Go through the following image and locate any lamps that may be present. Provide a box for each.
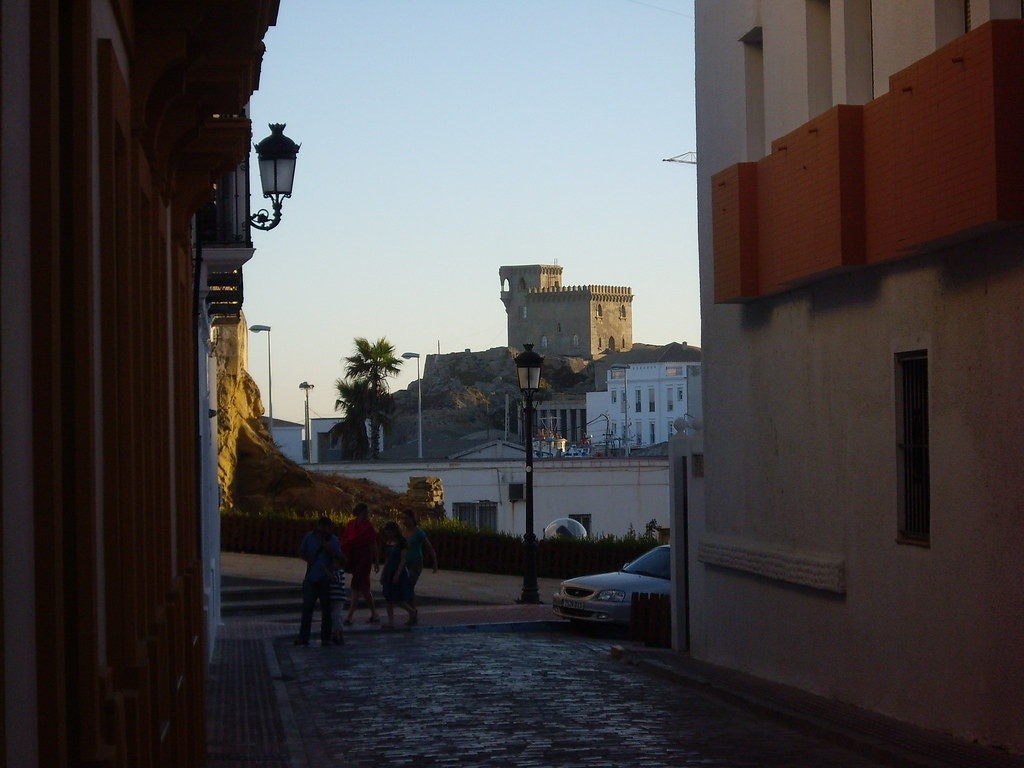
[250,122,300,231]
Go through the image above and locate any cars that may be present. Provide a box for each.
[552,543,670,639]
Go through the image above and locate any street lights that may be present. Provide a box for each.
[248,324,274,439]
[299,381,315,463]
[400,352,422,459]
[514,342,544,602]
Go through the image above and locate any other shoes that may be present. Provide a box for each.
[404,619,418,625]
[410,610,417,619]
[322,639,333,646]
[331,635,344,645]
[294,636,309,645]
[367,615,381,623]
[344,618,353,626]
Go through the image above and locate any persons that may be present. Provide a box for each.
[320,556,352,646]
[379,509,437,629]
[292,516,341,646]
[341,503,379,627]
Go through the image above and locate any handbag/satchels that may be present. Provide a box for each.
[423,551,432,569]
